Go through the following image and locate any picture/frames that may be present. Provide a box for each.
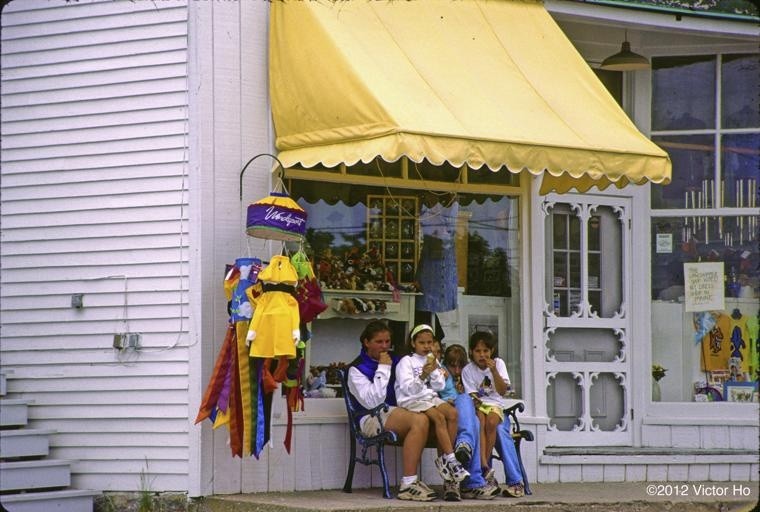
[723,382,759,403]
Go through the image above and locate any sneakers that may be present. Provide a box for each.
[396,481,438,502]
[461,485,501,500]
[483,467,498,487]
[434,440,473,481]
[443,482,462,501]
[502,482,526,498]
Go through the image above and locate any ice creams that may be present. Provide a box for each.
[427,353,435,363]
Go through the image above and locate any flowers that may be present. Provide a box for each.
[652,363,668,382]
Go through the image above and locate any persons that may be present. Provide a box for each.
[431,338,501,500]
[443,344,470,500]
[495,413,525,497]
[394,325,471,483]
[461,331,511,480]
[345,321,461,502]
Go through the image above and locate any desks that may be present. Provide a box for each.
[305,288,423,389]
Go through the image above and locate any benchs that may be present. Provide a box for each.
[337,368,534,499]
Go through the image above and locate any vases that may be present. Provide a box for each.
[652,383,660,401]
